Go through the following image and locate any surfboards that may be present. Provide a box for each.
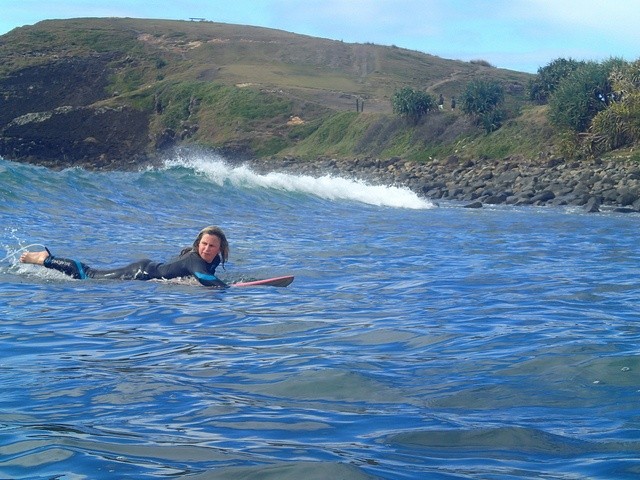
[229,276,295,287]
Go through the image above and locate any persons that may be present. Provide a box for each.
[20,225,233,290]
